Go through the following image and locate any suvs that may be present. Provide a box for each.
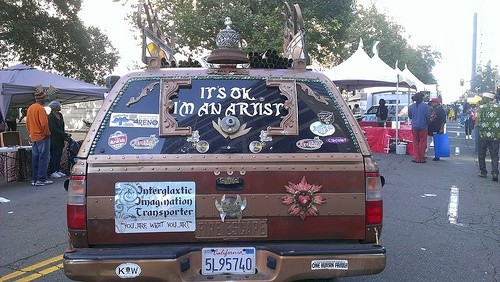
[61,68,387,282]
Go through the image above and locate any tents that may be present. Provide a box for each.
[0,63,116,184]
[319,37,439,99]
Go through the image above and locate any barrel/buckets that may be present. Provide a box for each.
[396,145,407,155]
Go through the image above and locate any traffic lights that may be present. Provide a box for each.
[460,78,465,86]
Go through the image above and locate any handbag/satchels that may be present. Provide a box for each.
[434,132,450,156]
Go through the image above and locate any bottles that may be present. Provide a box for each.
[383,121,386,128]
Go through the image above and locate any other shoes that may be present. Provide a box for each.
[433,157,439,161]
[466,135,468,139]
[468,135,472,139]
[478,170,487,178]
[32,181,44,185]
[492,174,498,181]
[411,159,426,163]
[36,180,53,184]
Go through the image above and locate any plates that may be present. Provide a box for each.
[310,121,336,138]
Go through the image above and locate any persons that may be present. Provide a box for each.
[444,102,463,121]
[427,97,447,136]
[48,101,71,179]
[474,91,491,154]
[25,85,54,186]
[464,101,473,140]
[377,99,388,125]
[408,91,430,164]
[476,89,500,181]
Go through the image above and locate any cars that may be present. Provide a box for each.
[362,104,410,124]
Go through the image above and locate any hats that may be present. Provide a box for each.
[35,89,46,101]
[430,98,439,102]
[49,101,60,109]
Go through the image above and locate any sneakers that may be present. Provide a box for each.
[56,171,66,176]
[50,172,61,177]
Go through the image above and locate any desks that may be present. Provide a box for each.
[358,121,414,155]
[0,145,33,183]
